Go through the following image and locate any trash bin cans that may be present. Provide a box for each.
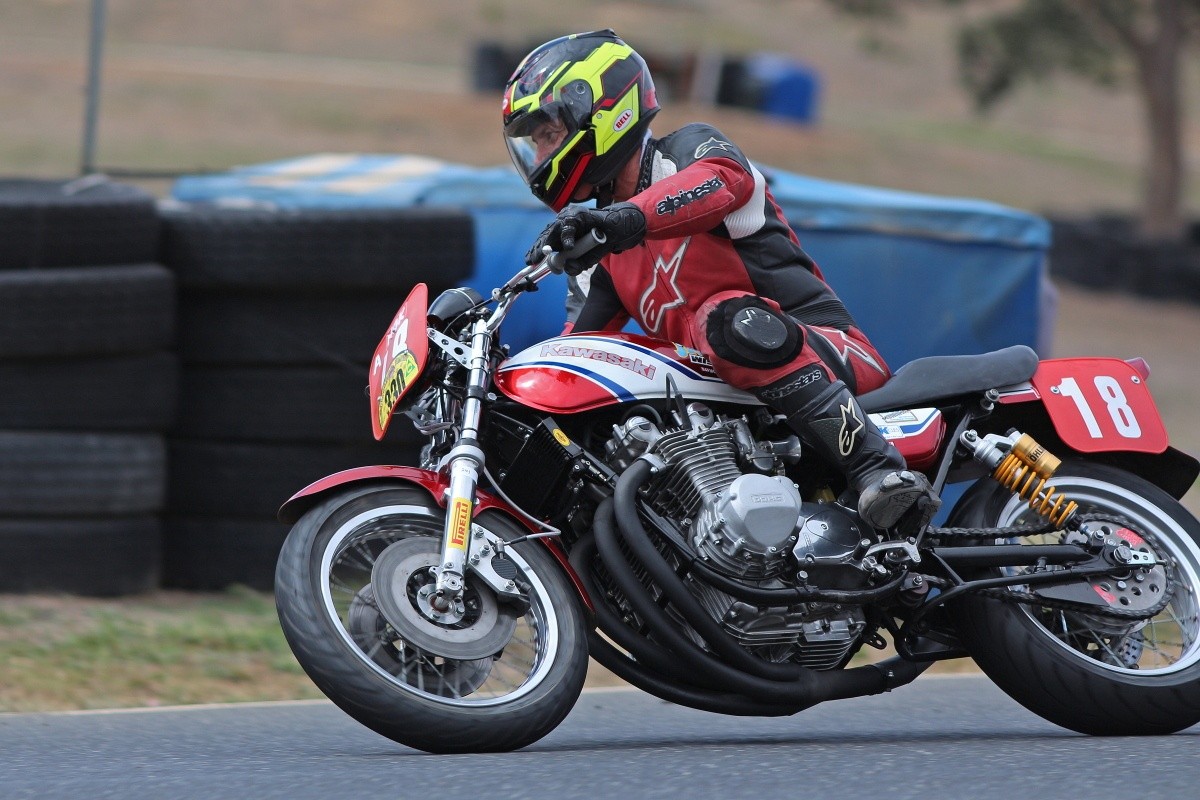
[720,53,815,118]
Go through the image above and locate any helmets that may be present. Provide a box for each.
[502,29,661,213]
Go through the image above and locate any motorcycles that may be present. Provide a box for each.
[274,226,1200,753]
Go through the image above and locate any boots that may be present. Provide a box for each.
[788,380,942,531]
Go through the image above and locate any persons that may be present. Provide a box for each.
[500,26,943,531]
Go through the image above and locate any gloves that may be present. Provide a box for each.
[525,201,645,276]
